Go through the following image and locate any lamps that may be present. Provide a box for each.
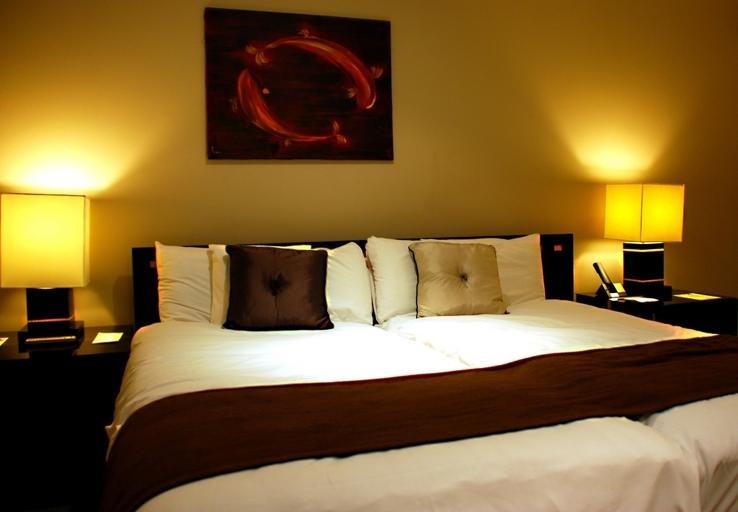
[603,183,685,301]
[1,193,90,343]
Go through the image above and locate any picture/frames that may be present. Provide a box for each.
[205,6,393,161]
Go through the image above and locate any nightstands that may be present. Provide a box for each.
[0,325,133,372]
[574,288,738,336]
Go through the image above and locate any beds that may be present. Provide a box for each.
[106,236,737,511]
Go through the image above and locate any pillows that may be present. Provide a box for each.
[208,242,373,327]
[407,241,510,318]
[365,233,546,324]
[155,239,312,323]
[221,245,335,330]
